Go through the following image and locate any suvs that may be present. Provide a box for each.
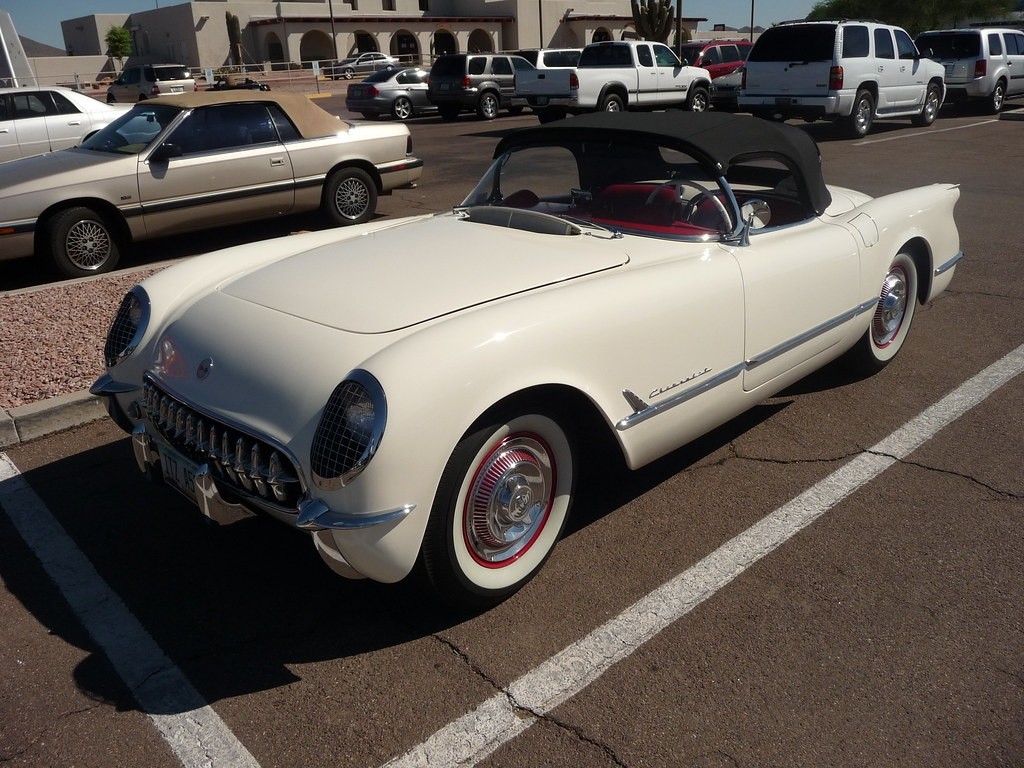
[668,38,755,106]
[735,16,948,138]
[105,62,199,103]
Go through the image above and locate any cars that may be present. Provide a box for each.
[204,77,272,92]
[0,86,164,164]
[344,65,440,120]
[323,52,400,81]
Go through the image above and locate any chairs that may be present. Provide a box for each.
[696,194,805,230]
[593,185,680,224]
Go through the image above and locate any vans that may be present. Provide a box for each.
[425,51,536,120]
[914,27,1024,116]
[513,45,584,69]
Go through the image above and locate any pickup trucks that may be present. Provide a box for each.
[512,40,714,125]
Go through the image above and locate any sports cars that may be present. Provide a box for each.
[1,81,427,279]
[87,108,966,613]
